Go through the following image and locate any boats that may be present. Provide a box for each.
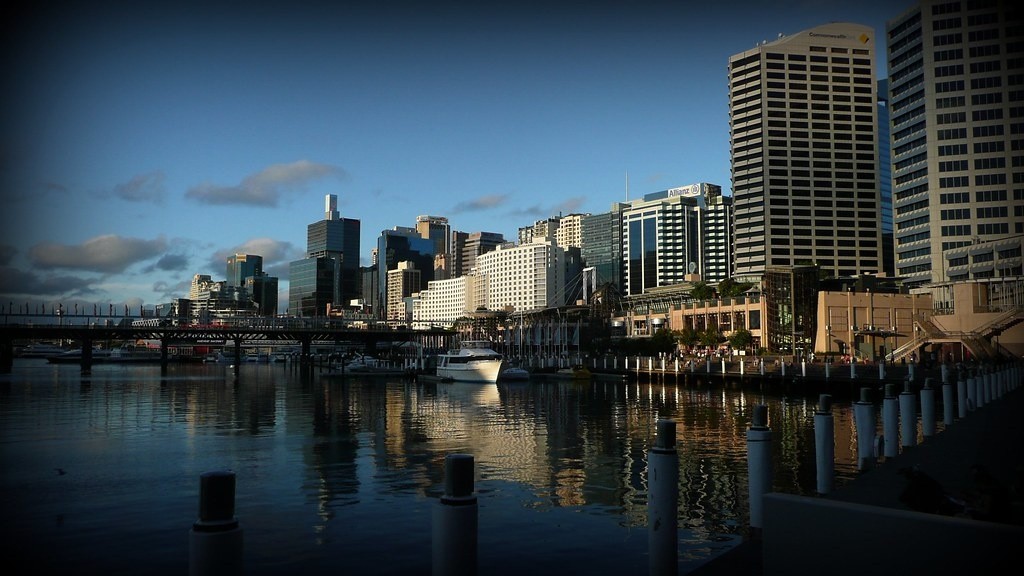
[247,352,289,362]
[47,348,119,364]
[502,367,530,379]
[436,341,502,383]
[557,364,591,378]
